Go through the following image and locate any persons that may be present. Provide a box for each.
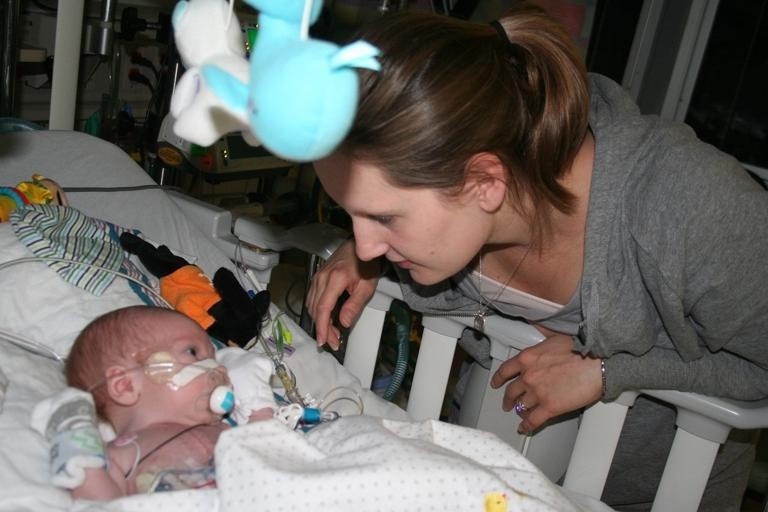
[37,304,281,503]
[299,1,765,510]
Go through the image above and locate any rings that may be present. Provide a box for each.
[512,398,529,418]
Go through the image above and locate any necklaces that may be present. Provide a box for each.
[471,241,533,341]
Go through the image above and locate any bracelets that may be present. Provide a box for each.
[599,354,607,398]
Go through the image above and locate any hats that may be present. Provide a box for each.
[10,202,125,298]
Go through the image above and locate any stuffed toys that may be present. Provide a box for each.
[167,0,262,151]
[201,0,386,165]
[0,173,68,226]
[120,231,273,350]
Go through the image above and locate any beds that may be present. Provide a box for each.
[0,121,768,511]
